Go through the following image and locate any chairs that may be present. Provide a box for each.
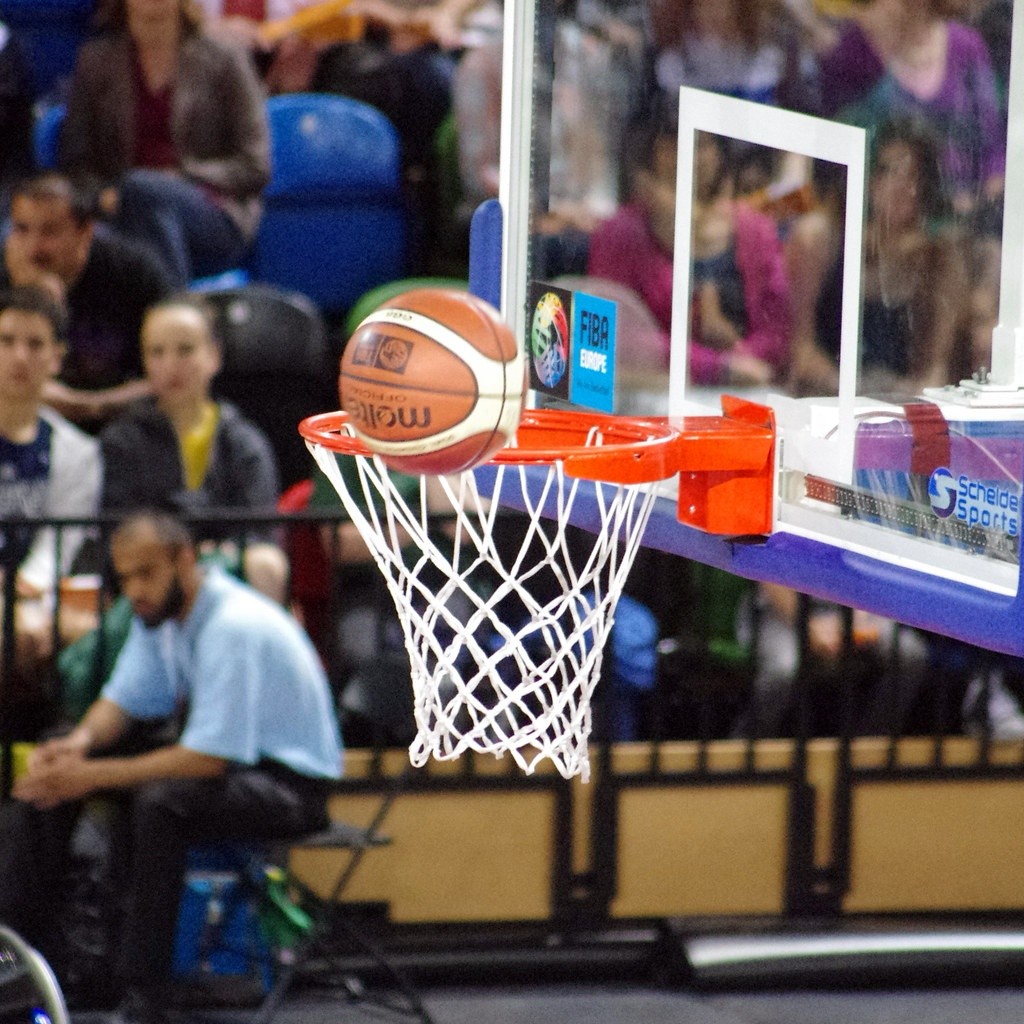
[206,289,324,488]
[175,652,463,1024]
[251,94,405,323]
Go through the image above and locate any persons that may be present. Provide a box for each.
[0,0,1006,1023]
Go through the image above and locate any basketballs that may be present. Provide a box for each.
[340,286,523,471]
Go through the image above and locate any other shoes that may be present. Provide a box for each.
[170,975,263,1009]
[68,1010,126,1024]
